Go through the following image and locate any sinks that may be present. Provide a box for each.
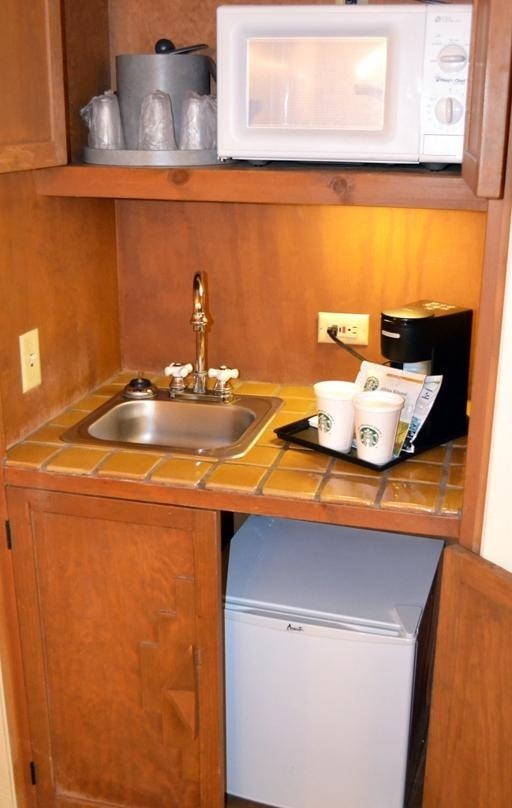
[61,387,283,460]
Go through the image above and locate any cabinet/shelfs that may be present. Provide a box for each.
[0,1,512,808]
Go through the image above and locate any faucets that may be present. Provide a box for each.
[190,270,209,397]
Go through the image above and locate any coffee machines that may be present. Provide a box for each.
[381,299,473,459]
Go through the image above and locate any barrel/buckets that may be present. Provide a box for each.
[115,43,208,152]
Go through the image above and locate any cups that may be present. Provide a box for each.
[88,96,124,149]
[313,380,360,454]
[352,389,405,465]
[137,96,176,150]
[180,98,212,150]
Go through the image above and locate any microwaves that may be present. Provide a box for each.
[215,4,473,165]
